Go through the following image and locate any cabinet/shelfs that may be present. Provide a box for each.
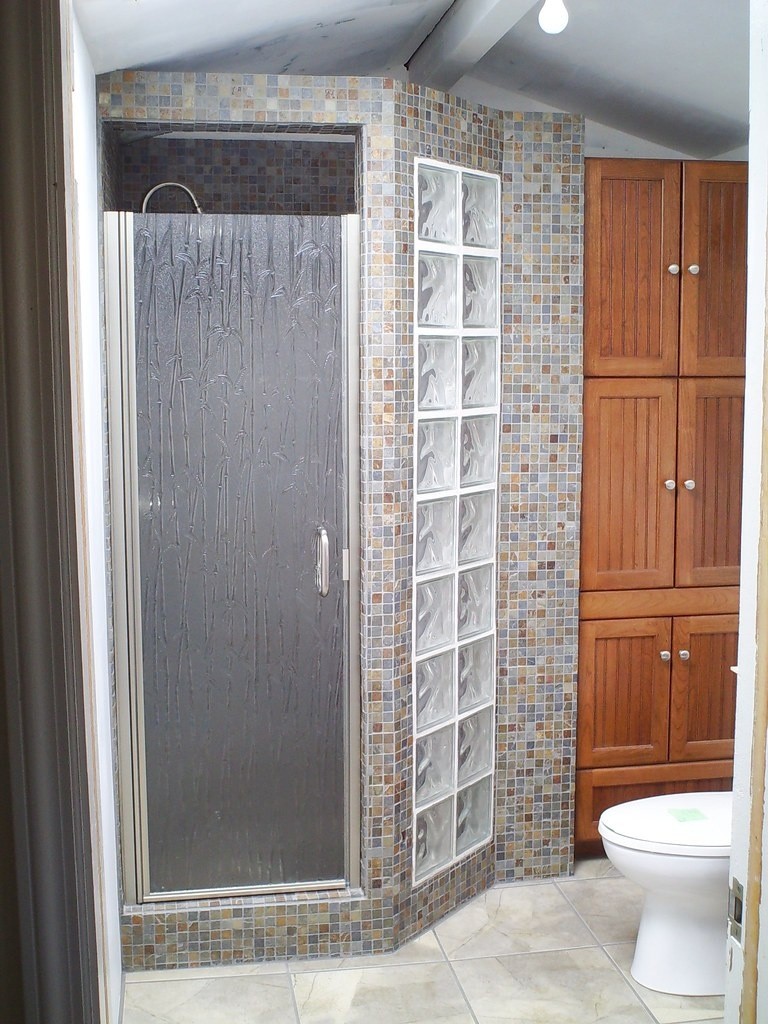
[573,158,749,862]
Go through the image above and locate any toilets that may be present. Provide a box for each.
[599,790,730,997]
